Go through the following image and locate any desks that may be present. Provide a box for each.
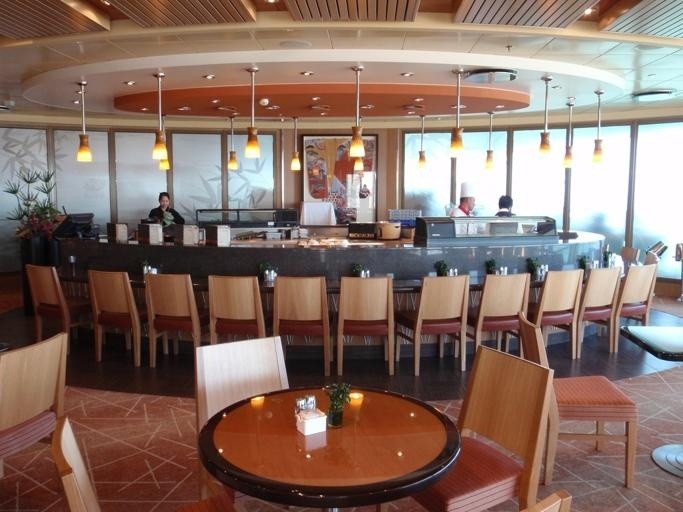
[197,385,462,512]
[620,325,683,478]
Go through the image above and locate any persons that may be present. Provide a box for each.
[450,192,477,234]
[149,192,185,225]
[489,195,518,234]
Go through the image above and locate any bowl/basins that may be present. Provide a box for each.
[521,224,536,233]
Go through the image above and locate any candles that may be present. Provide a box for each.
[251,396,265,405]
[347,392,364,406]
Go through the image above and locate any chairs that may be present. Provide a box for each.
[376,345,555,512]
[194,335,289,504]
[514,491,573,512]
[0,332,68,479]
[51,415,238,512]
[518,311,640,490]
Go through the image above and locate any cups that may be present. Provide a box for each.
[295,393,322,419]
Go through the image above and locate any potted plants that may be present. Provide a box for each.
[321,382,353,428]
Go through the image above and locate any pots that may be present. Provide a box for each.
[376,220,403,239]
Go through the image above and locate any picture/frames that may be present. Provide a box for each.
[300,133,378,227]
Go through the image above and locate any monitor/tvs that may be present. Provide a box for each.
[54,213,94,238]
[279,210,297,223]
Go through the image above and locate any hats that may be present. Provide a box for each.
[460,180,477,199]
